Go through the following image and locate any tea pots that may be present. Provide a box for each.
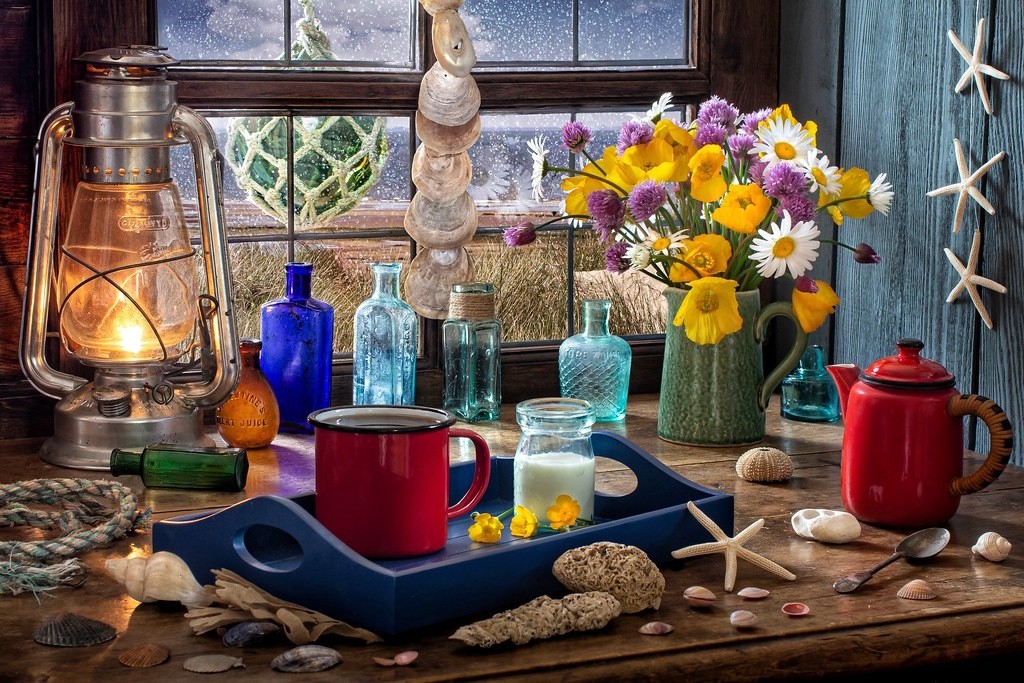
[826,339,1013,526]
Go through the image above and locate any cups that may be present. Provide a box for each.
[307,405,489,560]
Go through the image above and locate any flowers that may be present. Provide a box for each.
[510,506,554,539]
[468,509,514,543]
[545,494,593,533]
[502,91,895,346]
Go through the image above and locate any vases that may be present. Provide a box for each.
[438,282,502,421]
[557,300,634,422]
[656,287,809,448]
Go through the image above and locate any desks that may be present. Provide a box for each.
[0,395,1024,683]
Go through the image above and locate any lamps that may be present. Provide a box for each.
[17,44,243,472]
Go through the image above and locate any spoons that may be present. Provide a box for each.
[833,528,950,592]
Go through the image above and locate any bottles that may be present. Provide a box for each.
[261,262,334,435]
[514,398,595,532]
[558,300,631,420]
[110,443,248,490]
[780,345,841,421]
[443,283,502,422]
[352,264,417,404]
[215,339,280,449]
